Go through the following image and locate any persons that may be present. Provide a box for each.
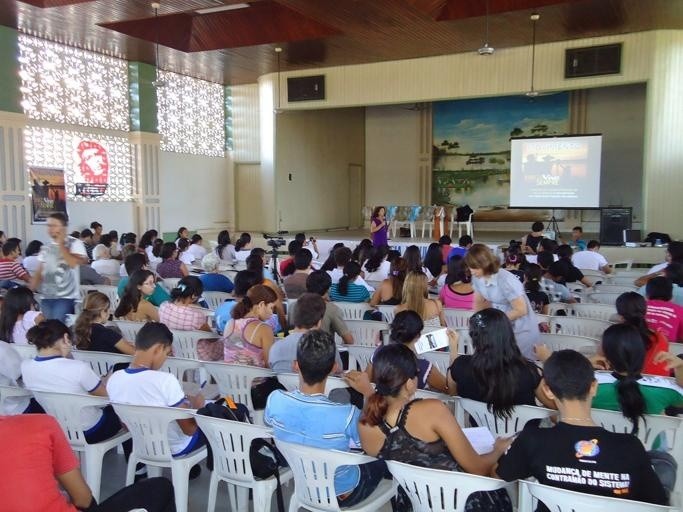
[77,141,109,193]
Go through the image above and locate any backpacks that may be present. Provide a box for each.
[363,310,389,340]
[196,397,280,480]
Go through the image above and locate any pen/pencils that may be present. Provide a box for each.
[508,431,521,439]
[198,380,207,393]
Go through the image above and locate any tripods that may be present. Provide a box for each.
[269,254,284,283]
[544,215,562,238]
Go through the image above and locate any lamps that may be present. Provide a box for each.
[526,15,541,98]
[477,1,495,57]
[273,47,285,115]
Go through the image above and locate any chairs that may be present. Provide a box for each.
[370,203,474,240]
[0,239,683,512]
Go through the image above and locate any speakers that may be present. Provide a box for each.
[599,206,632,246]
[287,75,325,102]
[564,42,622,78]
